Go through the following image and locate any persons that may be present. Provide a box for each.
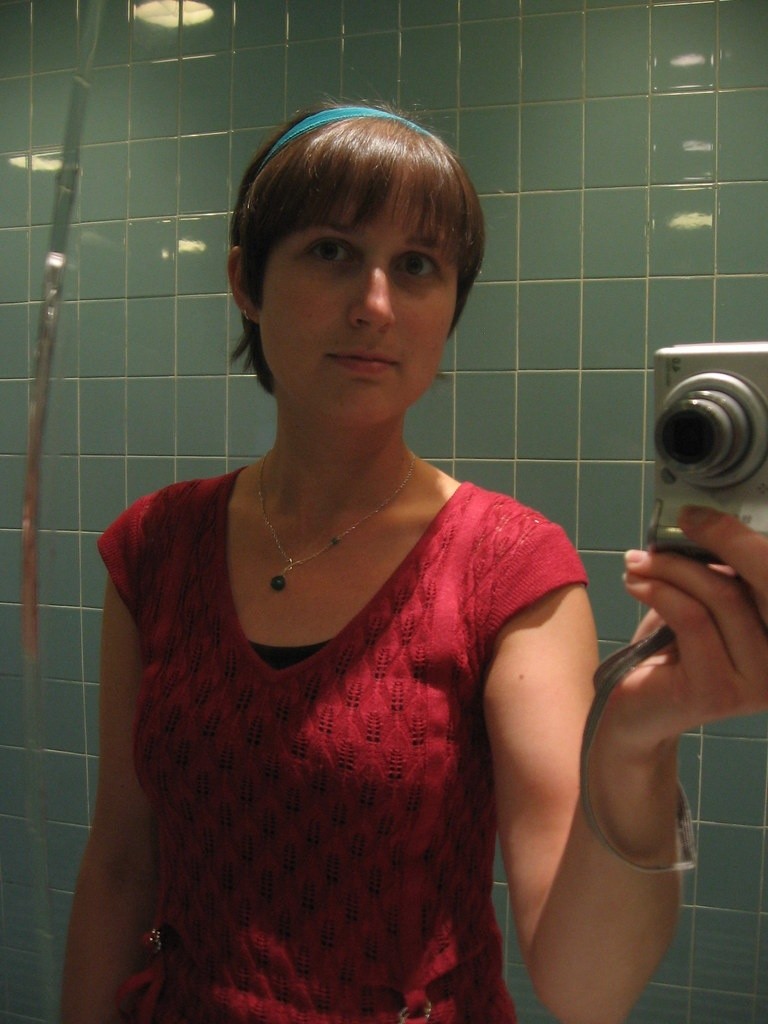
[62,96,766,1021]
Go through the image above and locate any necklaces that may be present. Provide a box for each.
[253,444,417,591]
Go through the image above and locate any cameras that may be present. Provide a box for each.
[645,340,768,552]
